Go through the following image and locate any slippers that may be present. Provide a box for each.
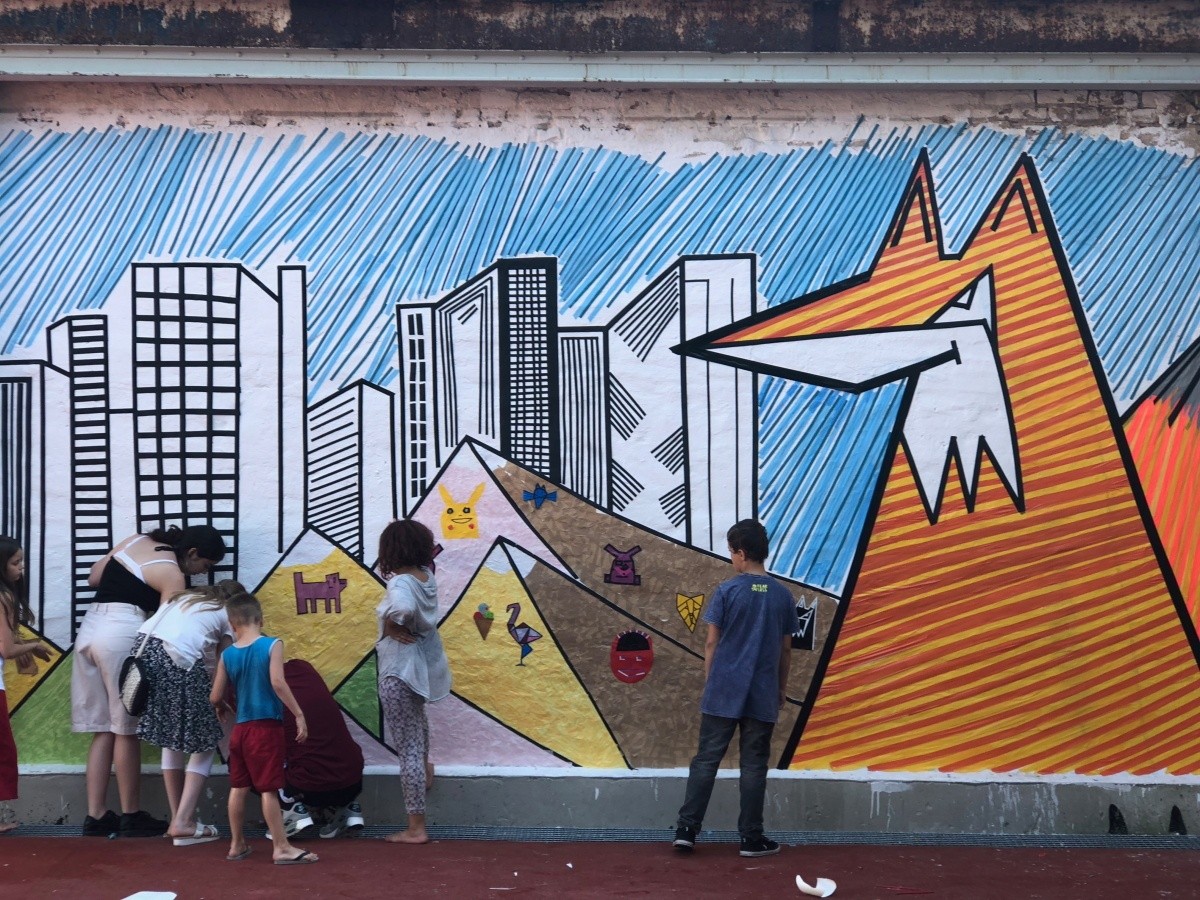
[227,845,252,860]
[173,822,220,846]
[275,850,317,864]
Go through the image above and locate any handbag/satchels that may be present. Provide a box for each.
[118,656,151,717]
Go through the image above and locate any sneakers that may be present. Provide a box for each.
[319,801,364,839]
[739,834,780,856]
[82,809,120,836]
[672,826,695,847]
[266,802,314,840]
[120,810,170,837]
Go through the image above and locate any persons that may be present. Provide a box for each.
[672,519,800,857]
[132,579,246,846]
[209,594,319,865]
[70,525,227,839]
[0,535,54,831]
[374,520,451,844]
[261,659,365,841]
